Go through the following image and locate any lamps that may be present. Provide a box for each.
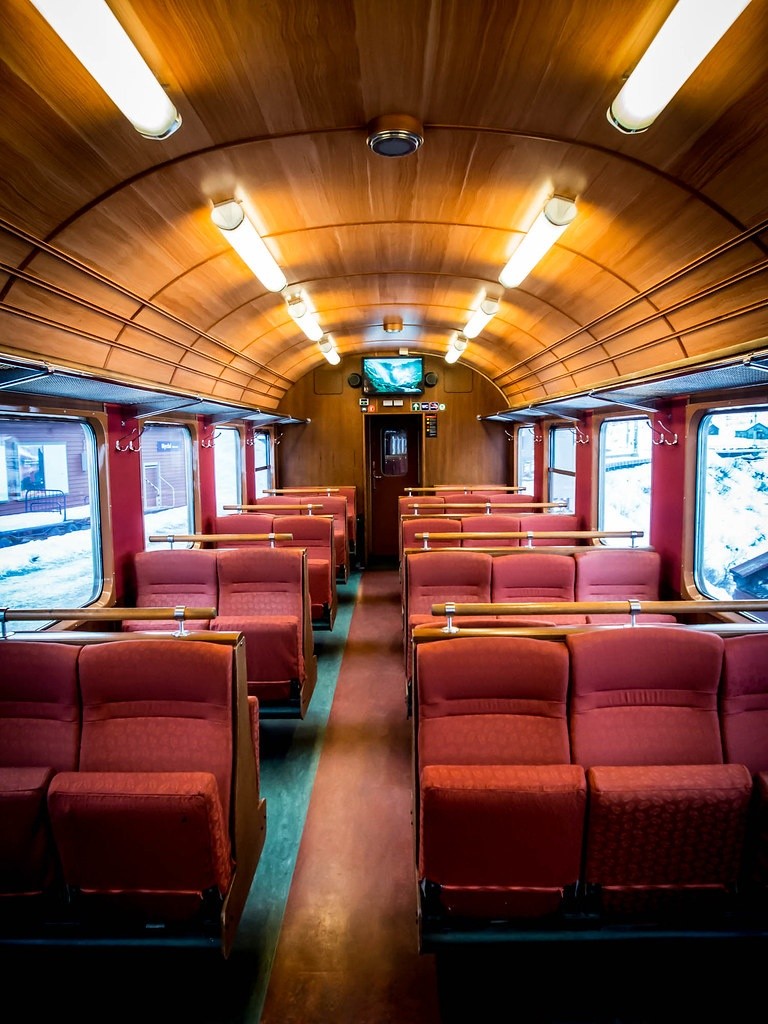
[29,0,183,141]
[288,298,323,341]
[498,194,577,289]
[606,0,752,135]
[319,339,341,366]
[445,336,467,364]
[211,202,288,293]
[463,296,499,339]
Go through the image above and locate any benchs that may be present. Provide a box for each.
[0,484,357,959]
[397,485,768,955]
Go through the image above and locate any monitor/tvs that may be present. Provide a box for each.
[361,356,424,395]
